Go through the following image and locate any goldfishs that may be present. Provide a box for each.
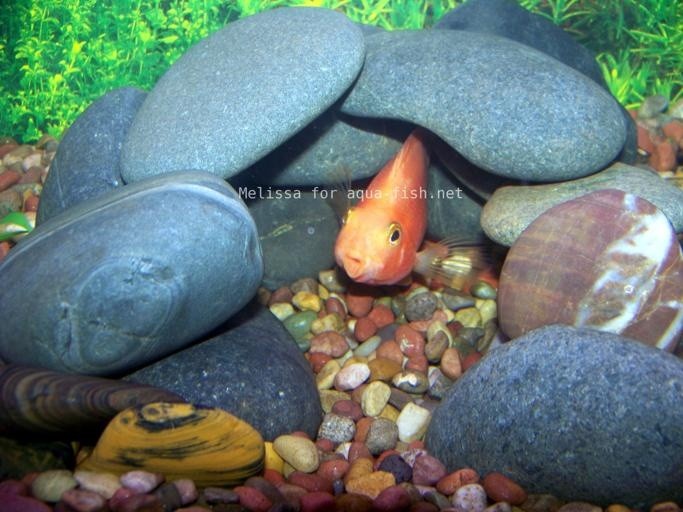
[317,126,508,292]
